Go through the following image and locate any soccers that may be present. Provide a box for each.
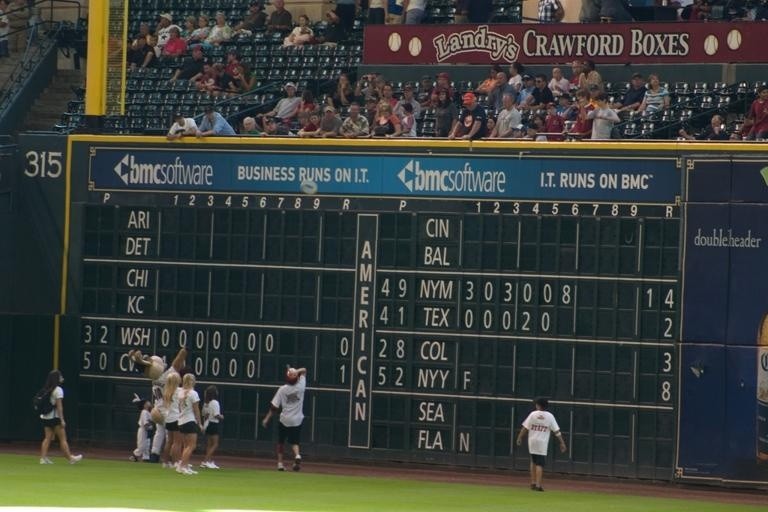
[727,30,743,50]
[704,35,719,55]
[408,38,421,56]
[388,33,402,51]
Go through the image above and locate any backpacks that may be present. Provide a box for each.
[33,386,54,415]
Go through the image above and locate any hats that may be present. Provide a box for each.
[160,10,309,88]
[48,370,64,384]
[280,69,584,119]
[287,368,297,379]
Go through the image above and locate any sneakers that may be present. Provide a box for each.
[151,455,160,463]
[142,459,150,462]
[69,453,83,465]
[39,458,54,465]
[278,462,288,472]
[162,460,198,476]
[293,455,302,472]
[128,455,139,462]
[200,461,219,469]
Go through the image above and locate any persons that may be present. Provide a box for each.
[129,11,232,72]
[171,45,258,102]
[261,366,307,471]
[37,369,83,465]
[167,107,235,140]
[234,0,356,53]
[579,0,767,23]
[244,73,435,137]
[609,73,670,135]
[515,396,567,491]
[678,87,767,141]
[368,0,494,24]
[0,0,31,65]
[538,0,564,24]
[122,346,227,474]
[430,60,619,142]
